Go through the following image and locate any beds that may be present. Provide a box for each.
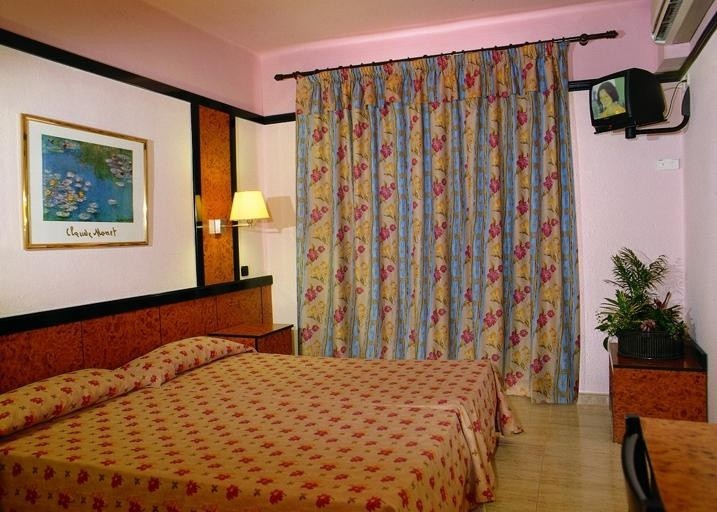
[0,335,520,511]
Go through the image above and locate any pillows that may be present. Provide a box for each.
[114,335,254,388]
[0,369,137,436]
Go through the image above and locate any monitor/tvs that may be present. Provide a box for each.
[590,68,665,126]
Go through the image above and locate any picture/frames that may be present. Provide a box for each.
[20,113,150,249]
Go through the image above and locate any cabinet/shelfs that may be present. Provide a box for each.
[607,342,708,445]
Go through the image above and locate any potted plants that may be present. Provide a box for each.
[594,245,688,360]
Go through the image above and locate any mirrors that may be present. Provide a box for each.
[191,102,241,286]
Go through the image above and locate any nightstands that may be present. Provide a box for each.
[210,323,294,354]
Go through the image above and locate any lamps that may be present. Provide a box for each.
[215,190,272,236]
[195,196,216,234]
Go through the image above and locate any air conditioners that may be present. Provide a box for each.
[650,0,715,44]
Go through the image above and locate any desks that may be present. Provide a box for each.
[641,416,717,512]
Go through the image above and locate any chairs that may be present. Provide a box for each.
[621,416,658,512]
